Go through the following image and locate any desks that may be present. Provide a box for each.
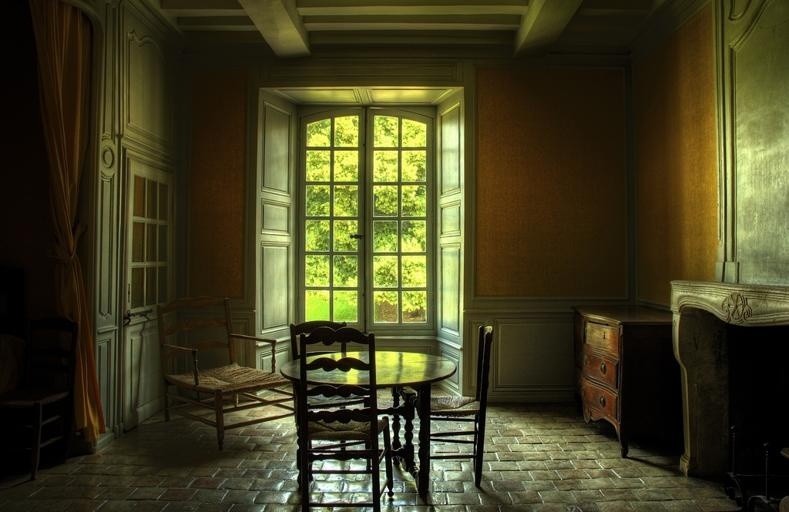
[280,350,457,496]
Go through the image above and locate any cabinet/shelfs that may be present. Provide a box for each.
[567,304,684,459]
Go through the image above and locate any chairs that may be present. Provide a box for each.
[156,295,297,450]
[289,320,495,512]
[1,310,77,480]
